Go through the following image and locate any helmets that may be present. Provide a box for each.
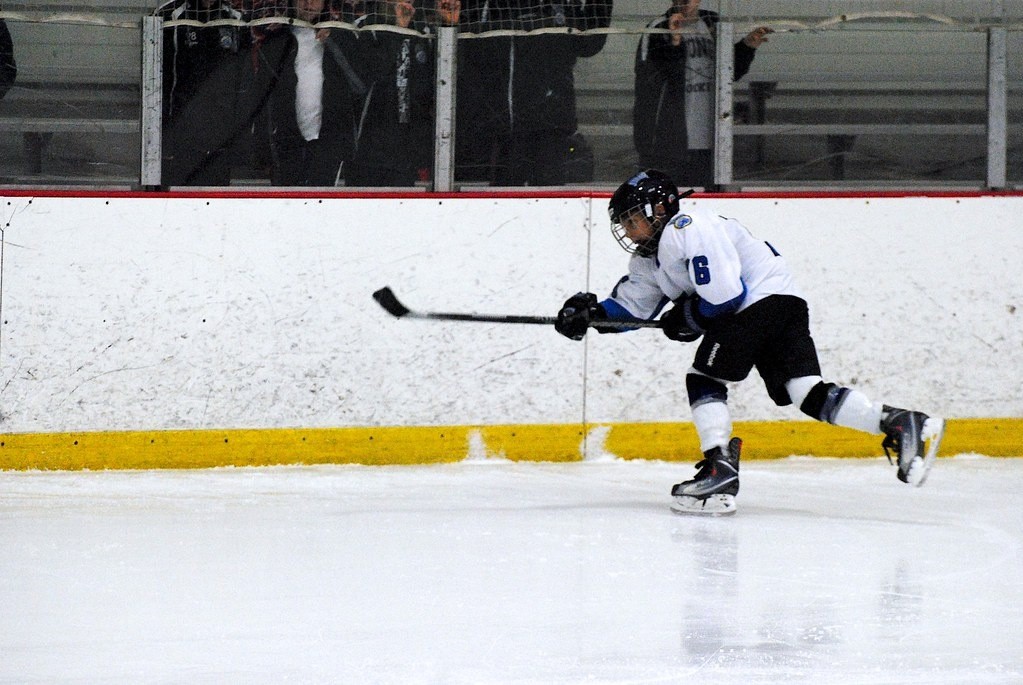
[609,169,680,259]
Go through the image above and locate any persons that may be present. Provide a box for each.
[633,1,773,185]
[146,0,614,188]
[553,167,945,518]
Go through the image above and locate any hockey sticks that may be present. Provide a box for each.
[371,285,663,329]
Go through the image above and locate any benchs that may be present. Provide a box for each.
[0,0,1023,137]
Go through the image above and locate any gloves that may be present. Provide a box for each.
[661,292,706,342]
[556,293,597,341]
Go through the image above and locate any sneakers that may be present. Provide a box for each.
[880,404,946,487]
[671,438,743,516]
